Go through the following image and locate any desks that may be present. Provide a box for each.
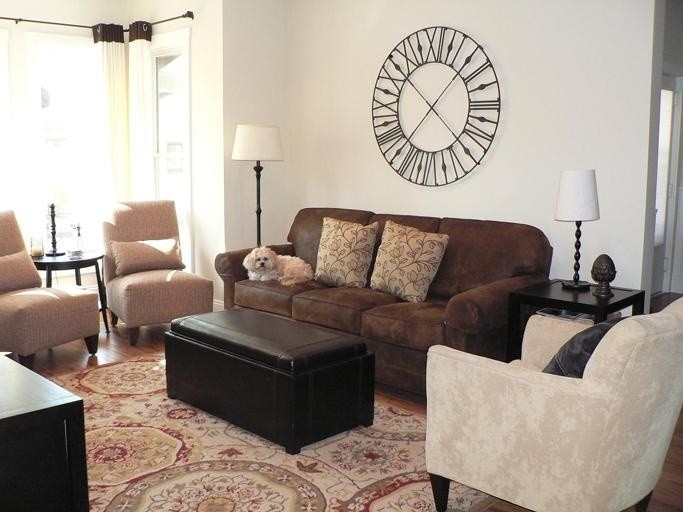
[505,274,645,365]
[162,307,374,457]
[32,248,112,335]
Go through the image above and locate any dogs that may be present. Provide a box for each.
[242,245,315,286]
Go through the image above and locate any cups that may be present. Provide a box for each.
[31,235,44,257]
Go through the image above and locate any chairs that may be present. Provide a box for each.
[421,292,682,510]
[0,202,99,367]
[95,196,215,350]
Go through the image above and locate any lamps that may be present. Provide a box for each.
[555,164,602,291]
[229,121,284,247]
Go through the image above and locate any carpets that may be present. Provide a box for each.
[40,344,511,511]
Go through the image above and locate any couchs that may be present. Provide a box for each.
[215,202,551,405]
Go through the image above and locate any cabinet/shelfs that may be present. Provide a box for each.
[0,350,93,512]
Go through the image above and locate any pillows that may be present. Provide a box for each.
[370,216,450,302]
[0,247,42,293]
[543,316,627,379]
[109,237,185,274]
[312,216,381,289]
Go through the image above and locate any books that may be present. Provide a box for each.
[536,307,583,322]
